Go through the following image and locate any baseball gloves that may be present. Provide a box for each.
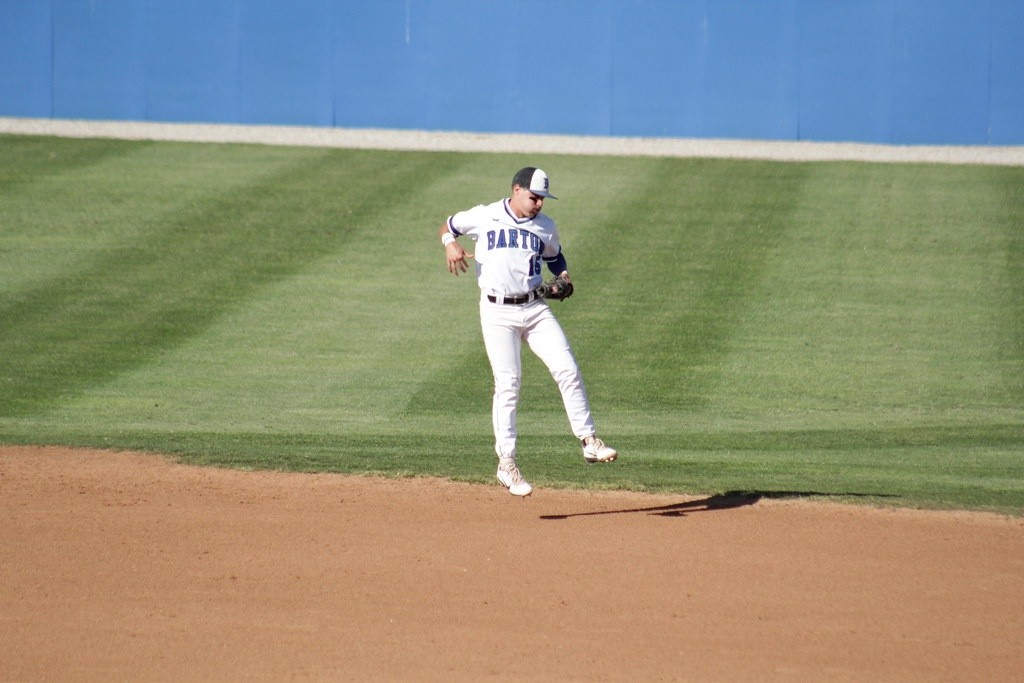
[538,277,574,302]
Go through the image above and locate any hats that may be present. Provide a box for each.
[512,167,560,201]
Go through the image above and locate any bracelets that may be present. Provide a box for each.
[441,232,455,246]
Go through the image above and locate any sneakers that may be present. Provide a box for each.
[496,463,533,498]
[582,438,618,464]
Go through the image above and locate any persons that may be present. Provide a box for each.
[439,166,618,498]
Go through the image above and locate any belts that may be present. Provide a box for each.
[488,291,539,304]
